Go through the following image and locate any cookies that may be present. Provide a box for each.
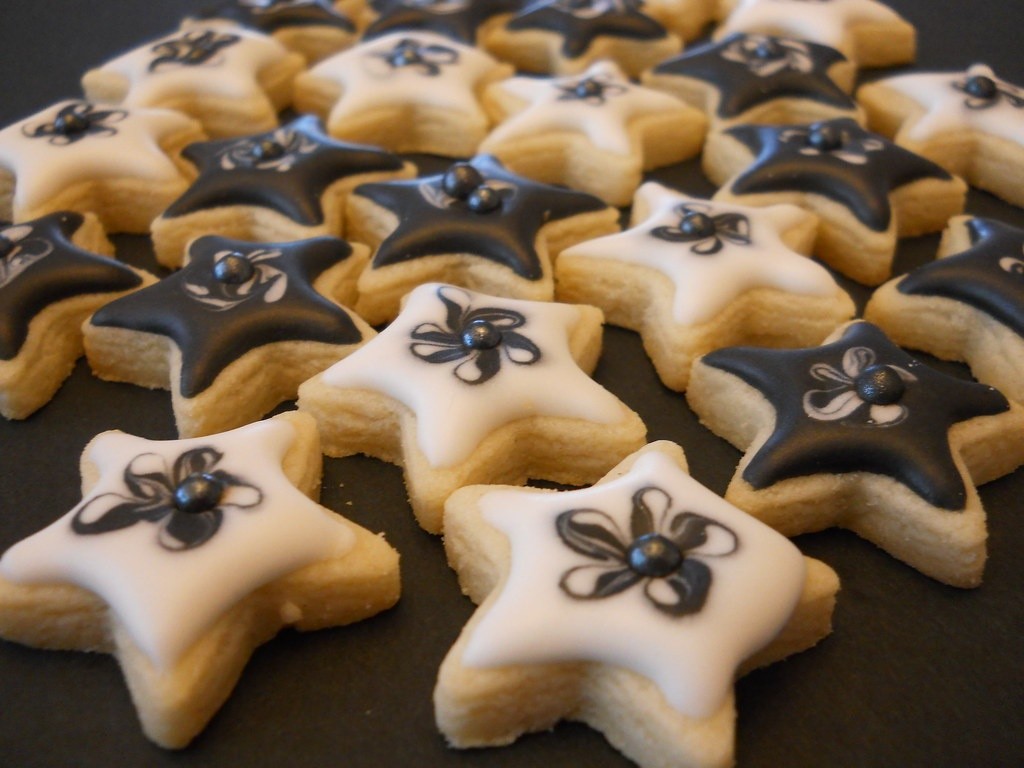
[0,0,1024,768]
